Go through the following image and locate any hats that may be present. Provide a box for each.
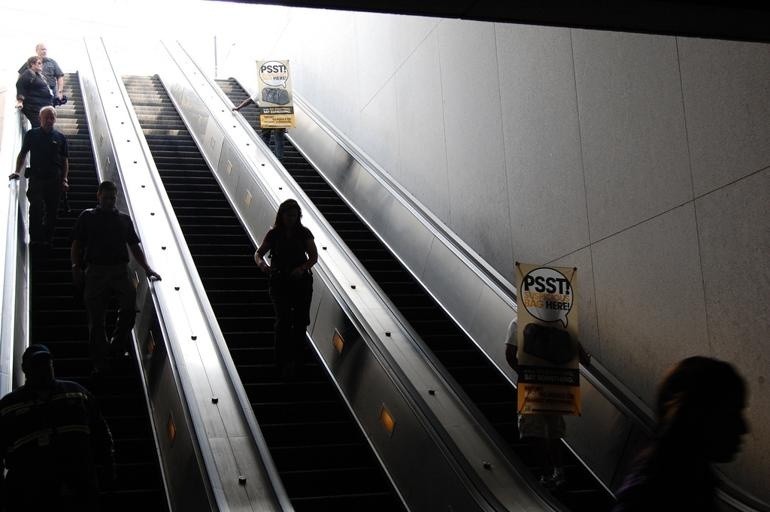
[21,340,56,366]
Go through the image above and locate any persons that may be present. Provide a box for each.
[9,106,70,244]
[254,199,317,366]
[0,344,113,512]
[616,356,749,512]
[232,97,284,161]
[15,43,64,128]
[71,181,162,357]
[505,316,591,486]
